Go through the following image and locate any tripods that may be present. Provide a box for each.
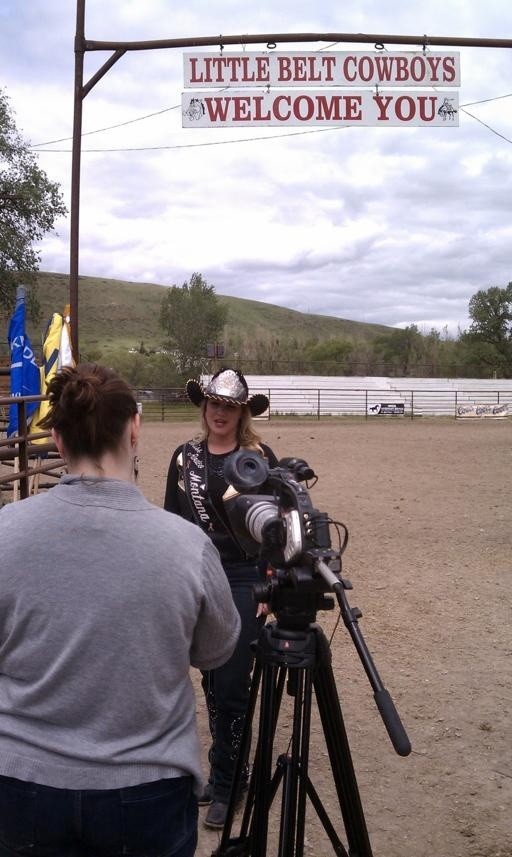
[212,548,412,857]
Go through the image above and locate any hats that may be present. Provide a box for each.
[186,367,269,417]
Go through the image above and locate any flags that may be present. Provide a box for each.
[27,310,63,446]
[6,284,42,452]
[56,303,76,375]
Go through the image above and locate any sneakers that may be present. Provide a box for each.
[196,783,243,828]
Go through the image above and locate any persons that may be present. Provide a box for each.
[0,363,243,855]
[161,362,281,835]
